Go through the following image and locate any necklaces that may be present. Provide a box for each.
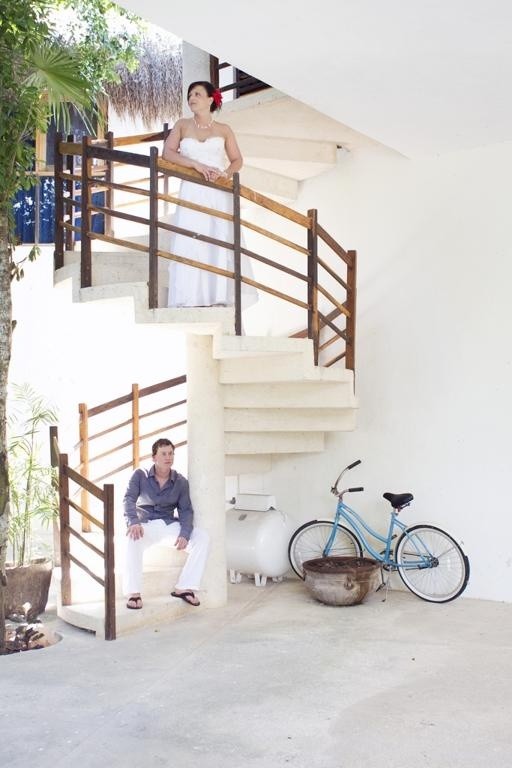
[192,117,213,131]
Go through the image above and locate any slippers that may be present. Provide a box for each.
[127,596,143,609]
[171,591,201,606]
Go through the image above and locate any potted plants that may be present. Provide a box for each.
[4,379,62,620]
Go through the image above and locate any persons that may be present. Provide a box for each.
[161,81,245,307]
[124,439,201,609]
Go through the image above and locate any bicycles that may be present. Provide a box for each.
[287,458,470,602]
